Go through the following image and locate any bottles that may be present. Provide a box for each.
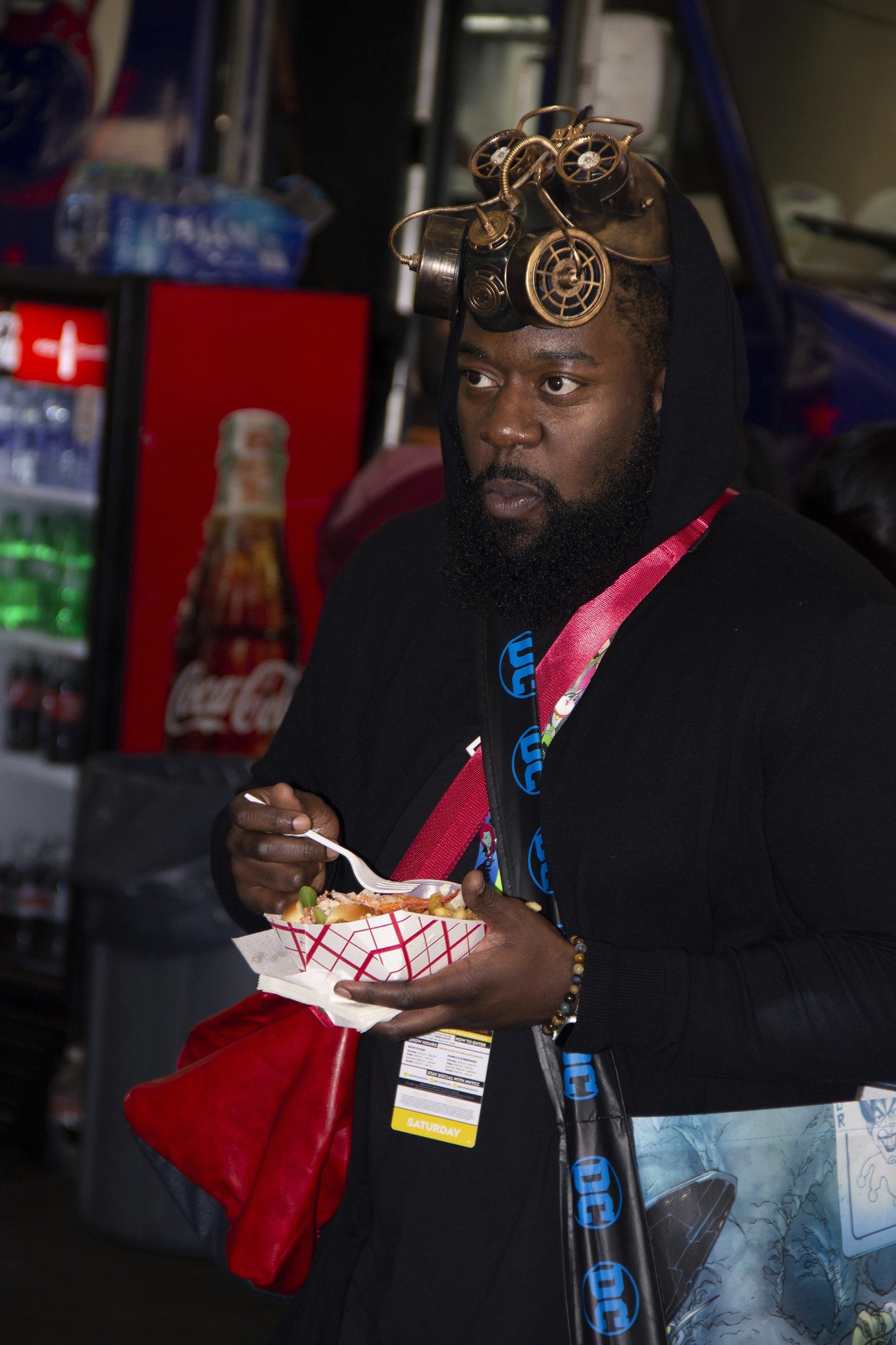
[164,408,304,757]
[1,383,104,1199]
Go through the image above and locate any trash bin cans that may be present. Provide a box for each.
[72,753,259,1254]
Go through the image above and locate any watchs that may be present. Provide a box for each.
[552,1000,580,1049]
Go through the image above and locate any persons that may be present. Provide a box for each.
[210,151,896,1345]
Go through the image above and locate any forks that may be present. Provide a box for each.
[244,793,420,893]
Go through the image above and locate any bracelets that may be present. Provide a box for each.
[541,933,587,1034]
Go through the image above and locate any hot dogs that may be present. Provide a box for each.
[279,886,428,924]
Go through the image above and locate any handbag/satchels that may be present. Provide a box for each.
[124,989,360,1296]
